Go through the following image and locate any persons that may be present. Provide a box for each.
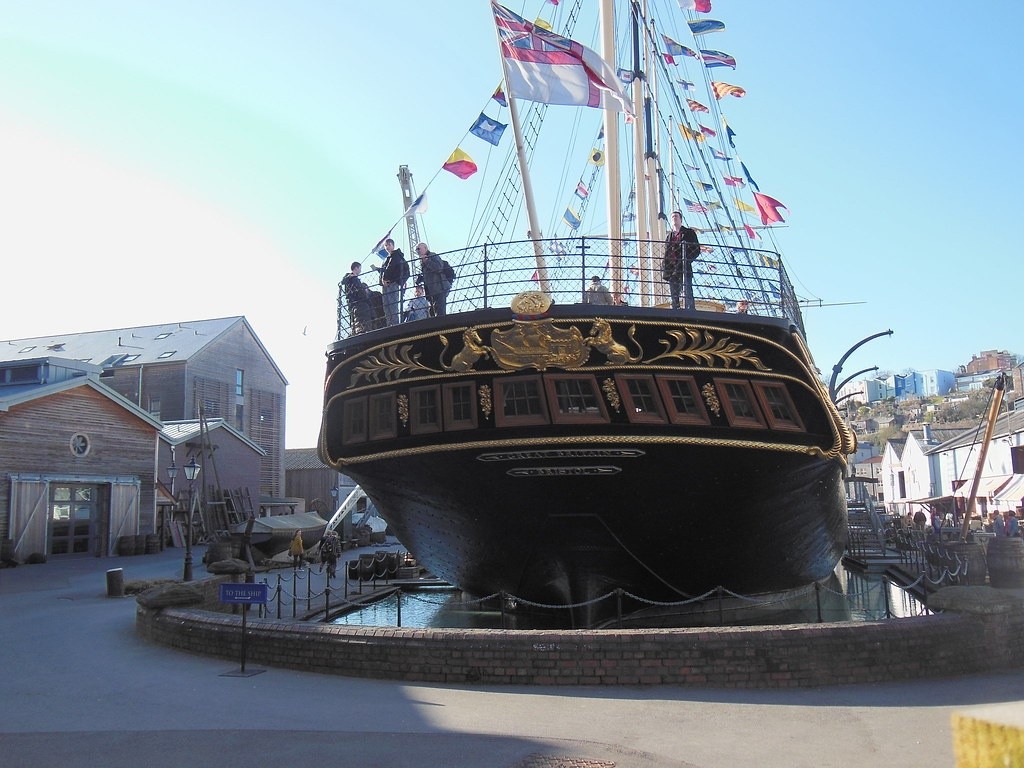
[292,528,304,571]
[376,239,404,326]
[406,286,429,321]
[586,276,613,305]
[902,508,940,542]
[994,510,1018,537]
[318,531,341,578]
[341,262,386,331]
[661,211,700,309]
[415,242,452,316]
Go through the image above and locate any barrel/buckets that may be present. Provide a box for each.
[120,533,161,557]
[208,542,232,567]
[946,544,986,585]
[985,537,1024,588]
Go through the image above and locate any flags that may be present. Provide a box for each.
[491,2,633,125]
[661,0,787,298]
[546,0,559,6]
[469,112,509,147]
[535,17,551,31]
[617,68,632,83]
[443,148,477,180]
[622,192,639,294]
[405,194,428,216]
[373,234,391,260]
[492,86,508,107]
[531,128,604,284]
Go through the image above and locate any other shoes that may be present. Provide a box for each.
[330,572,336,579]
[319,566,323,573]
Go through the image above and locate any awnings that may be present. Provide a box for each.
[889,474,1024,512]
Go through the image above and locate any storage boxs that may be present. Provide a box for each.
[398,566,419,579]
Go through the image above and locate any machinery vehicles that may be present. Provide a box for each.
[396,165,429,296]
[937,371,1013,544]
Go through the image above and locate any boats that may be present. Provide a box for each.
[228,511,329,555]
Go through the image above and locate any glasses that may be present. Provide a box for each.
[416,248,421,251]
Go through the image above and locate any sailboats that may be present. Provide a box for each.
[315,0,858,620]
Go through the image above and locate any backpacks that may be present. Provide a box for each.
[322,536,335,557]
[396,257,411,284]
[432,255,457,285]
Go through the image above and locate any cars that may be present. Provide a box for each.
[846,507,871,532]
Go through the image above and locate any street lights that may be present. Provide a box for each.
[182,454,202,581]
[330,486,338,514]
[166,460,182,522]
